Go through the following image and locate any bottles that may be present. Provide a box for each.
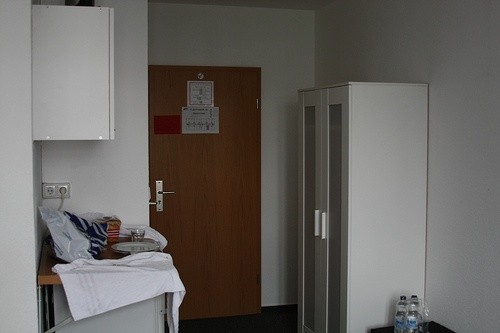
[394,296,408,333]
[406,296,420,333]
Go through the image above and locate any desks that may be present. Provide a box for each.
[39,233,183,333]
[371,321,457,333]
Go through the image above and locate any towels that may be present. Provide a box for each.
[51,251,187,333]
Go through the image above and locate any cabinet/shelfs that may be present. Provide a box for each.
[31,5,116,142]
[295,80,430,333]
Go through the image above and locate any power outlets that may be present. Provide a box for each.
[42,183,69,198]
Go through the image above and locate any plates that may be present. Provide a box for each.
[111,242,157,252]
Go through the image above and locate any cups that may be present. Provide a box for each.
[131,230,144,242]
